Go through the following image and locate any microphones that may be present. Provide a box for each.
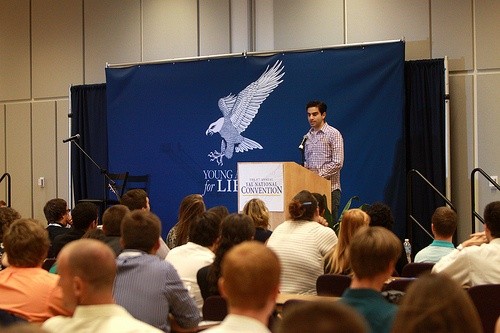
[299,135,308,150]
[63,133,81,143]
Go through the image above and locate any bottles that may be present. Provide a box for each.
[403,239,411,263]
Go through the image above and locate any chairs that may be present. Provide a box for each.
[0,247,499,333]
[77,172,149,224]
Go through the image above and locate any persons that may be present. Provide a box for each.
[431,201,500,289]
[324,203,395,277]
[112,210,201,333]
[164,194,273,320]
[41,238,165,333]
[0,206,74,325]
[196,240,281,333]
[413,206,461,264]
[241,190,338,296]
[273,226,403,333]
[392,271,481,333]
[42,189,171,274]
[302,100,344,226]
